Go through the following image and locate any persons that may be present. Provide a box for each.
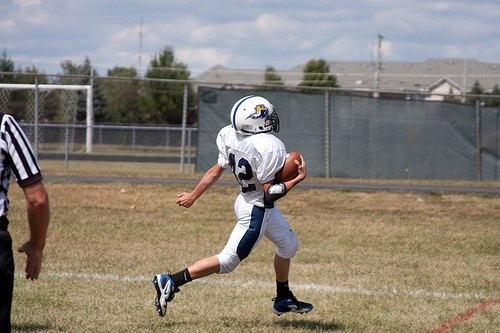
[0,113,50,333]
[153,95,314,317]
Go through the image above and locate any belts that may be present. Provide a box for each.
[0,217,8,231]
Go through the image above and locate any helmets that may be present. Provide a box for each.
[230,95,274,135]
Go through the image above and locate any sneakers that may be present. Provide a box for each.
[151,270,180,317]
[272,291,313,316]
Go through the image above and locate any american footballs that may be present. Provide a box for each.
[273,151,302,182]
